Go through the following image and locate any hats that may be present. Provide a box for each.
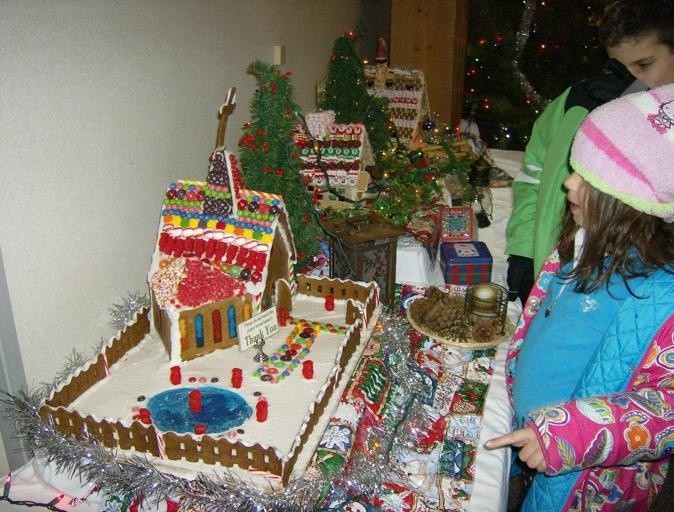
[569,83,674,223]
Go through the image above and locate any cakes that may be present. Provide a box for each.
[35,67,449,491]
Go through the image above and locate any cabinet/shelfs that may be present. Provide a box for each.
[389,2,468,133]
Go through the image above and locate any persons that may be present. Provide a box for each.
[501,57,655,300]
[481,76,674,512]
[598,0,674,92]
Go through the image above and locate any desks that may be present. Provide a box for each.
[2,140,525,512]
